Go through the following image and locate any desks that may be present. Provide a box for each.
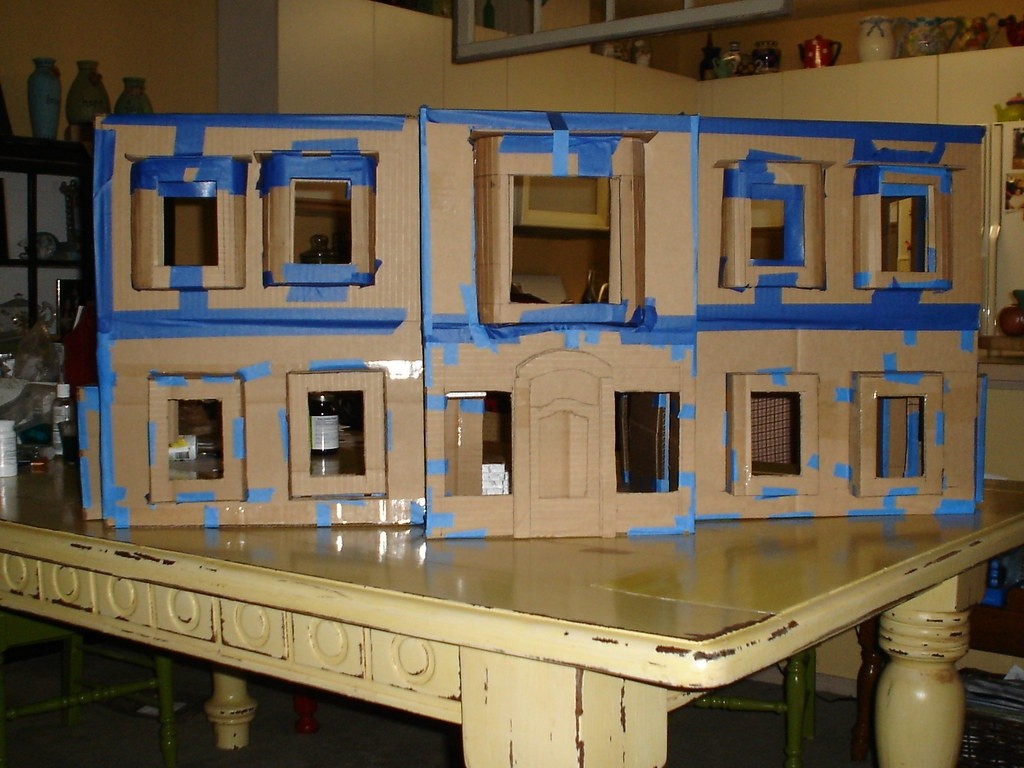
[0,451,1024,768]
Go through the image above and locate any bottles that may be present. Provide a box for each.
[0,420,17,477]
[52,384,76,456]
[66,59,107,123]
[700,47,721,80]
[309,234,331,264]
[114,76,153,114]
[310,396,340,453]
[28,57,61,139]
[722,41,743,77]
[752,40,780,74]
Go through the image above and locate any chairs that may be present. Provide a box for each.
[850,543,1024,763]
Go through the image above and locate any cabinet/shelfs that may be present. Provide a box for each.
[216,0,1024,125]
[979,363,1022,483]
[0,133,93,344]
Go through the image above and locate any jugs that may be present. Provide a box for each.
[902,13,1024,56]
[857,15,909,60]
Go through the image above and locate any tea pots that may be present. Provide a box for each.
[994,93,1024,122]
[798,35,841,68]
